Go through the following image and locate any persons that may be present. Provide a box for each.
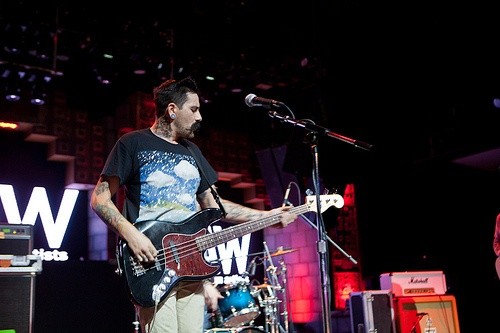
[202,223,263,329]
[90,77,297,333]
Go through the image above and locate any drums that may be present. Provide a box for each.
[203,328,236,333]
[237,327,268,333]
[213,284,261,328]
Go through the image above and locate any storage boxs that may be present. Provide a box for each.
[0,272,36,333]
[348,289,395,333]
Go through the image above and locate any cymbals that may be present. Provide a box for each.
[254,283,280,290]
[262,247,300,259]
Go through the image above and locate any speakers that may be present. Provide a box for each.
[391,295,461,333]
[0,272,33,333]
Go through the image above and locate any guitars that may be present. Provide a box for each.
[116,188,344,308]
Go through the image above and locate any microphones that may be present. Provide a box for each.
[242,256,256,279]
[244,93,283,108]
[282,186,291,207]
[416,313,428,316]
